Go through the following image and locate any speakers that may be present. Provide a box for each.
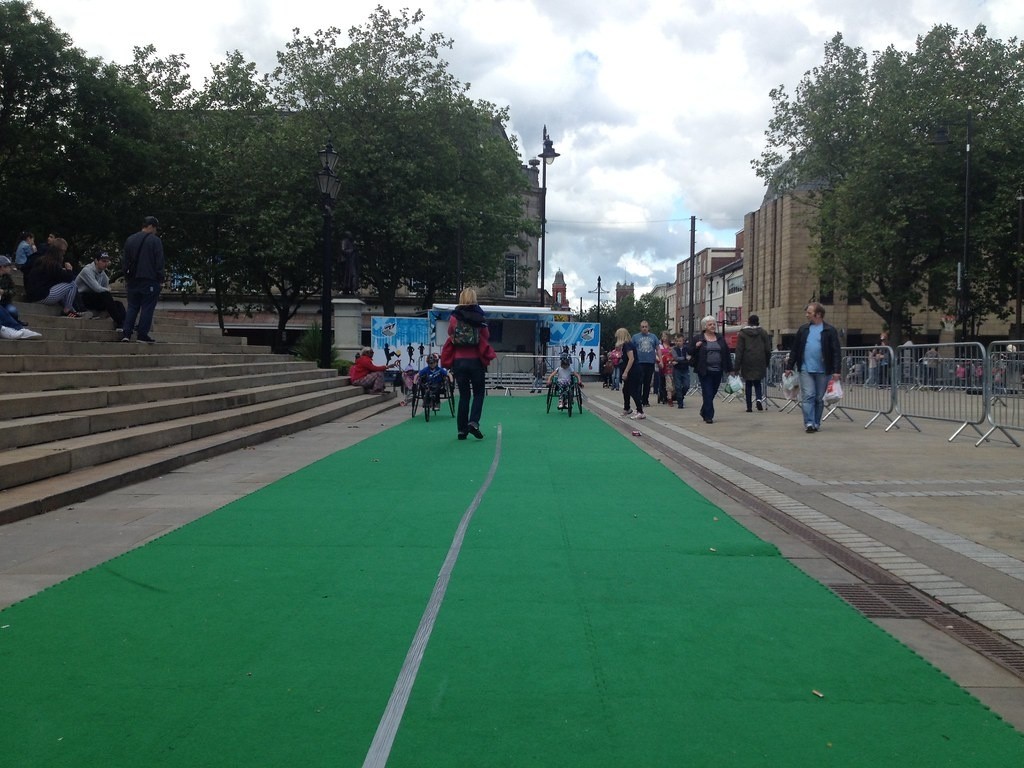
[539,327,550,343]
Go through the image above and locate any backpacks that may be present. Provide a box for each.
[451,317,480,344]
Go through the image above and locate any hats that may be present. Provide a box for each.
[0,255,11,267]
[95,252,113,261]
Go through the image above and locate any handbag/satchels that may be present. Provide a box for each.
[822,378,843,405]
[124,260,135,284]
[725,374,743,394]
[782,371,800,399]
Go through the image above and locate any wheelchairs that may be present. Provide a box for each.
[545,375,584,419]
[411,374,455,422]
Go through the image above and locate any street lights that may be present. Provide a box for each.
[537,121,563,306]
[314,137,343,368]
[927,102,974,359]
[587,275,611,323]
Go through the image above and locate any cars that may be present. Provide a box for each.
[729,353,737,372]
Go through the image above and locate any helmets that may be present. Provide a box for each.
[561,353,572,365]
[426,353,440,363]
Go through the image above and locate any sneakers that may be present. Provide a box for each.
[19,328,42,339]
[61,310,84,319]
[0,326,23,339]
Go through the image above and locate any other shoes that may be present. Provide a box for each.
[805,425,819,433]
[422,401,432,408]
[558,401,563,408]
[136,336,156,343]
[610,382,763,424]
[458,434,467,440]
[632,413,646,421]
[116,328,124,332]
[619,409,632,416]
[434,403,440,410]
[564,399,568,408]
[120,334,131,342]
[468,422,483,439]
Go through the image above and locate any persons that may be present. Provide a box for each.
[785,302,841,433]
[579,348,596,368]
[733,315,772,412]
[601,331,691,409]
[773,344,783,351]
[688,315,734,423]
[530,355,547,393]
[0,232,127,340]
[925,347,941,391]
[863,341,888,389]
[442,287,497,441]
[350,347,395,394]
[384,343,425,367]
[120,216,165,342]
[414,354,453,410]
[632,321,663,407]
[616,320,645,419]
[547,353,584,409]
